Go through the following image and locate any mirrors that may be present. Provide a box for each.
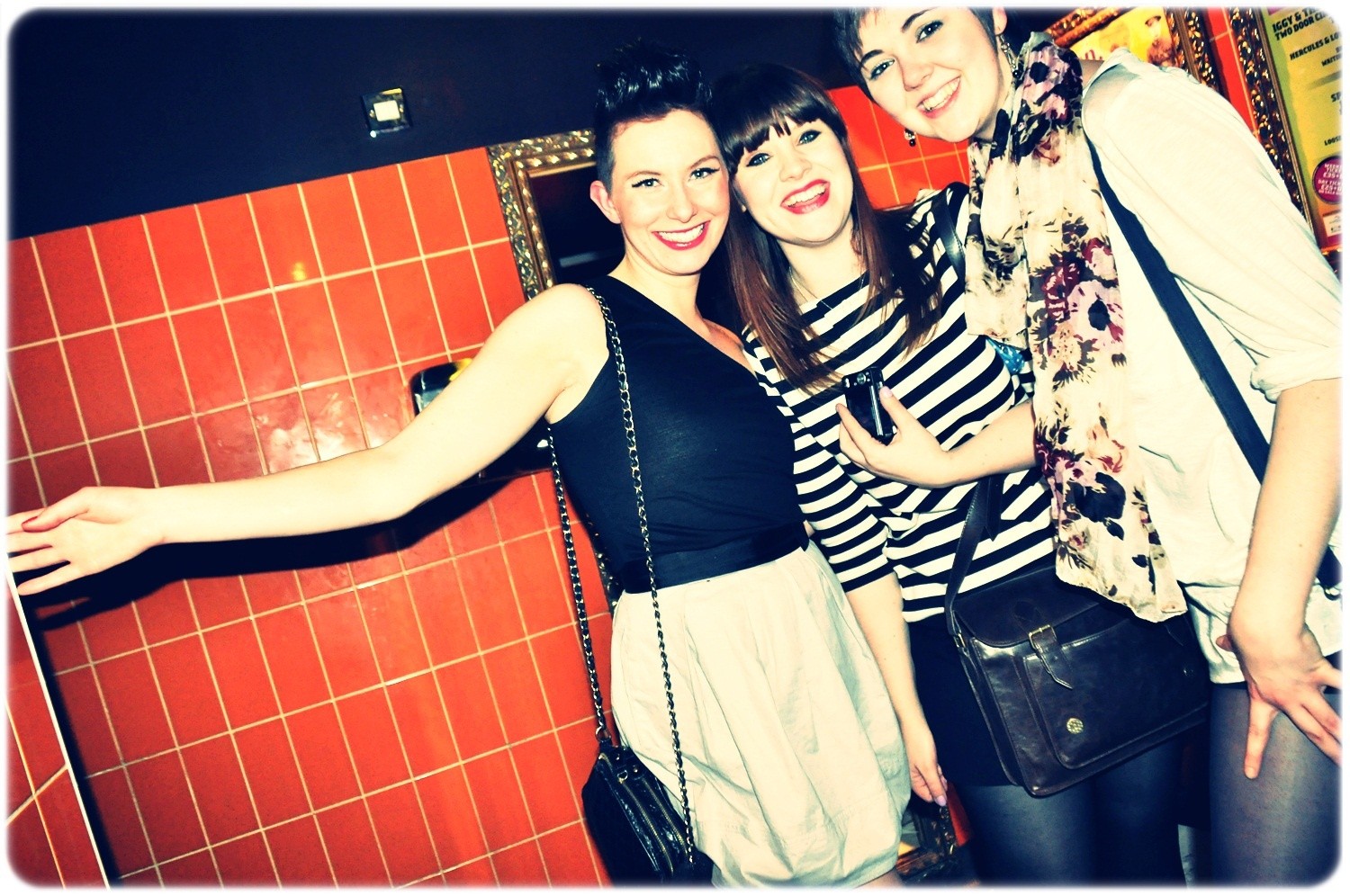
[489,126,623,310]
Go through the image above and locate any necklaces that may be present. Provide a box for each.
[797,275,867,313]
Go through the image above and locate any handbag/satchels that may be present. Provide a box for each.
[581,745,699,886]
[945,561,1211,799]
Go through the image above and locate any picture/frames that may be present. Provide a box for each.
[1043,8,1340,281]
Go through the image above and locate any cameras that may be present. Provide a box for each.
[841,366,897,445]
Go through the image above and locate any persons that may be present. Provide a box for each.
[705,7,1342,887]
[7,48,918,888]
